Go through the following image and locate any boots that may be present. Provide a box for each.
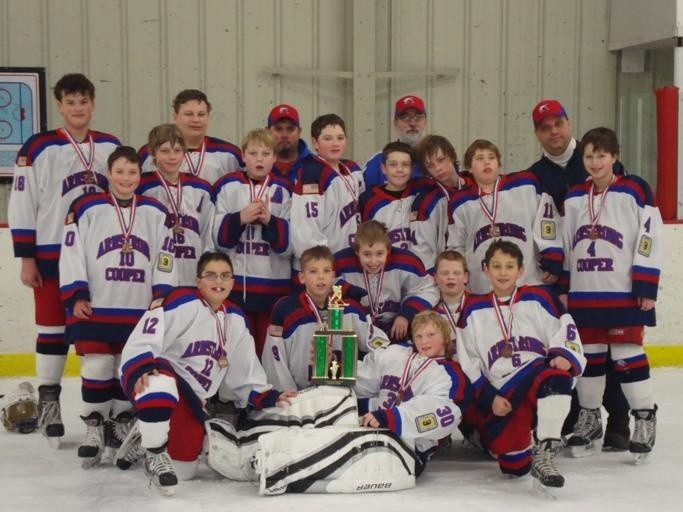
[39,384,66,438]
[567,401,658,453]
[531,428,566,487]
[78,411,180,486]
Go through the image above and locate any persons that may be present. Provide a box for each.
[205,311,462,492]
[455,236,584,498]
[7,73,663,464]
[113,252,290,487]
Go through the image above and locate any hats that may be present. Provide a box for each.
[393,97,426,117]
[533,101,566,129]
[267,104,300,128]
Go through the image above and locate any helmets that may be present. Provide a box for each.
[1,383,39,433]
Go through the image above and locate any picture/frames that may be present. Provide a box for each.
[0,65,48,184]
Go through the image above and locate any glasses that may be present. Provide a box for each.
[202,272,236,281]
[400,113,425,121]
